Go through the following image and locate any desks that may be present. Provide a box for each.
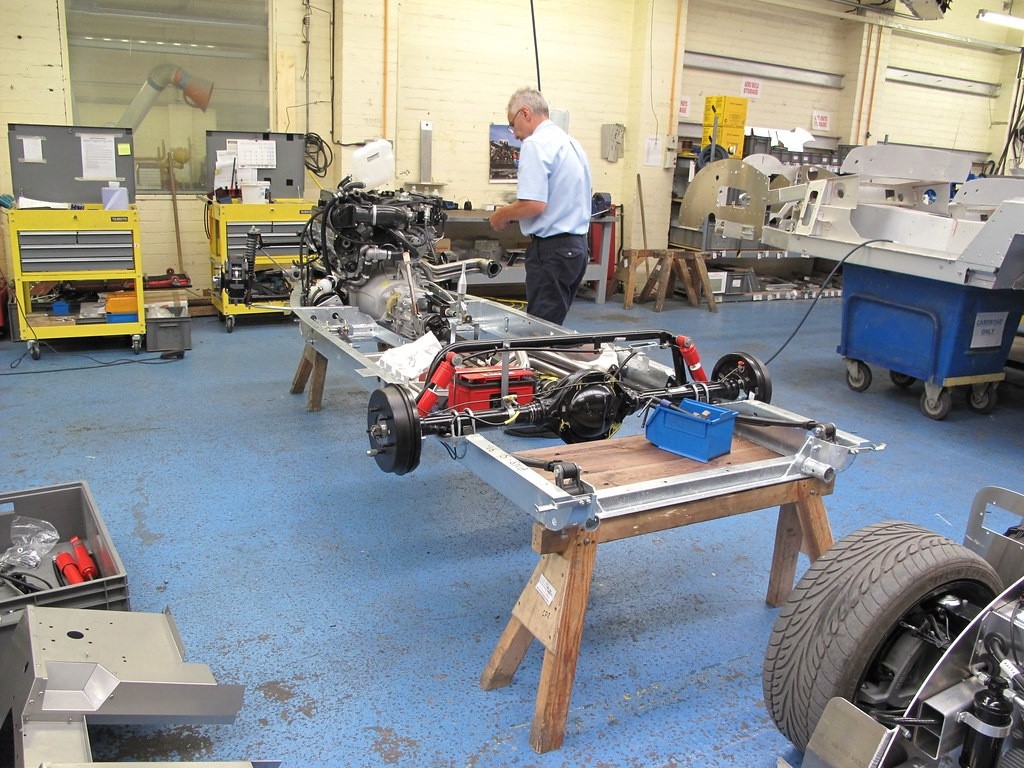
[442,209,620,304]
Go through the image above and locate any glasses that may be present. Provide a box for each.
[507,109,522,134]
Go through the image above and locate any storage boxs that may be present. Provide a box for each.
[744,135,863,166]
[646,398,739,463]
[673,267,749,295]
[669,217,788,250]
[701,96,746,160]
[7,291,64,342]
[104,292,138,323]
[0,481,131,631]
[435,238,451,252]
[52,300,69,315]
[143,288,193,351]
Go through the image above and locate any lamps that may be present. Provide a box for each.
[976,0,1024,31]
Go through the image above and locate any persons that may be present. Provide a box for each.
[489,89,591,326]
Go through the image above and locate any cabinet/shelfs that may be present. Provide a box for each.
[194,194,325,333]
[0,194,148,361]
